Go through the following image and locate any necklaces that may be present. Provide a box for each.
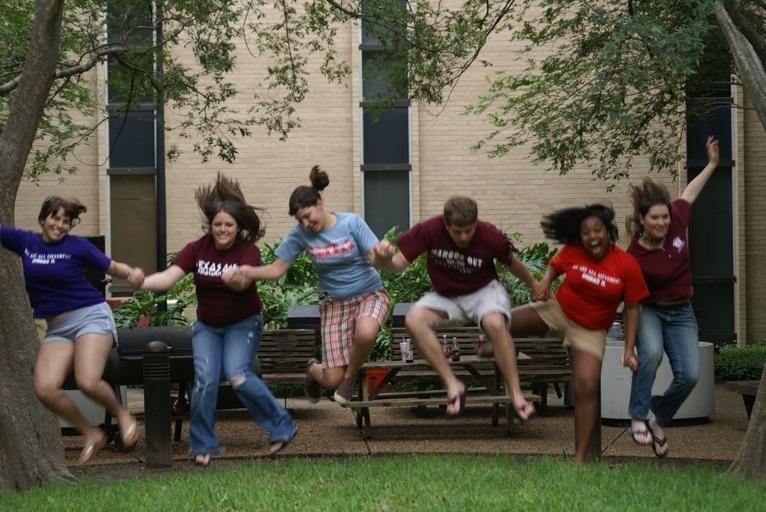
[638,232,664,243]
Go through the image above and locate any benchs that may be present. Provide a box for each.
[391,326,572,416]
[256,327,316,408]
[341,391,543,435]
[349,384,504,402]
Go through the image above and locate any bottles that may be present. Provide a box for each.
[405,334,489,363]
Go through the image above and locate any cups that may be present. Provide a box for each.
[400,342,409,362]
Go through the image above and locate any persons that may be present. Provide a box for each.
[237,164,396,406]
[0,196,145,465]
[478,202,650,465]
[128,171,298,467]
[379,197,544,421]
[621,135,720,457]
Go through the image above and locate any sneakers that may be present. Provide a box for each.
[304,358,319,403]
[333,378,353,405]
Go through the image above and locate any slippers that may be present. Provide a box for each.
[271,425,299,453]
[515,402,532,422]
[81,433,107,465]
[653,437,667,457]
[627,427,652,446]
[193,454,209,467]
[447,395,464,417]
[122,422,137,447]
